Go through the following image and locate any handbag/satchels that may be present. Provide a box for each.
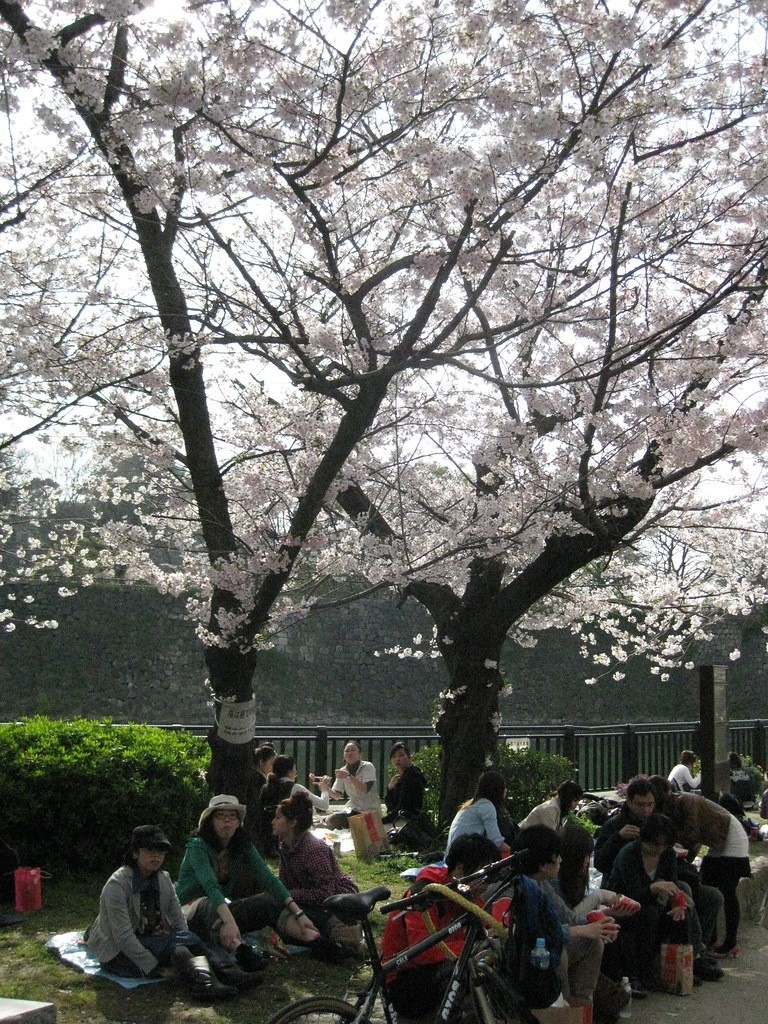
[659,942,694,996]
[529,997,592,1024]
[419,882,540,1024]
[4,868,52,912]
[388,808,444,852]
[347,809,389,861]
[583,867,603,896]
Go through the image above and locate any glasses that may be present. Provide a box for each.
[213,813,238,820]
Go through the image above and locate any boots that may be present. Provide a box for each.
[182,956,240,1006]
[212,959,262,991]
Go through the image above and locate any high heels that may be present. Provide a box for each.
[713,944,740,959]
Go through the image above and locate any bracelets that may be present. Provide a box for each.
[346,773,351,780]
[287,900,294,908]
[293,909,305,920]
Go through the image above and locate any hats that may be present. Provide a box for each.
[198,794,246,831]
[130,825,172,849]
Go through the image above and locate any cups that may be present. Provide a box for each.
[314,777,322,789]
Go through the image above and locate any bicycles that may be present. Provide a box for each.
[260,846,541,1024]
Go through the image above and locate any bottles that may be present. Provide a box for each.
[619,977,632,1018]
[531,938,550,968]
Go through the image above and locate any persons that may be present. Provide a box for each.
[247,739,428,859]
[380,750,768,1024]
[86,790,363,1000]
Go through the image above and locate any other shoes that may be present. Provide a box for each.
[308,935,347,966]
[613,966,648,998]
[693,955,724,986]
[235,943,269,971]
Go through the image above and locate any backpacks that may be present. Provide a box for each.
[508,852,564,1009]
